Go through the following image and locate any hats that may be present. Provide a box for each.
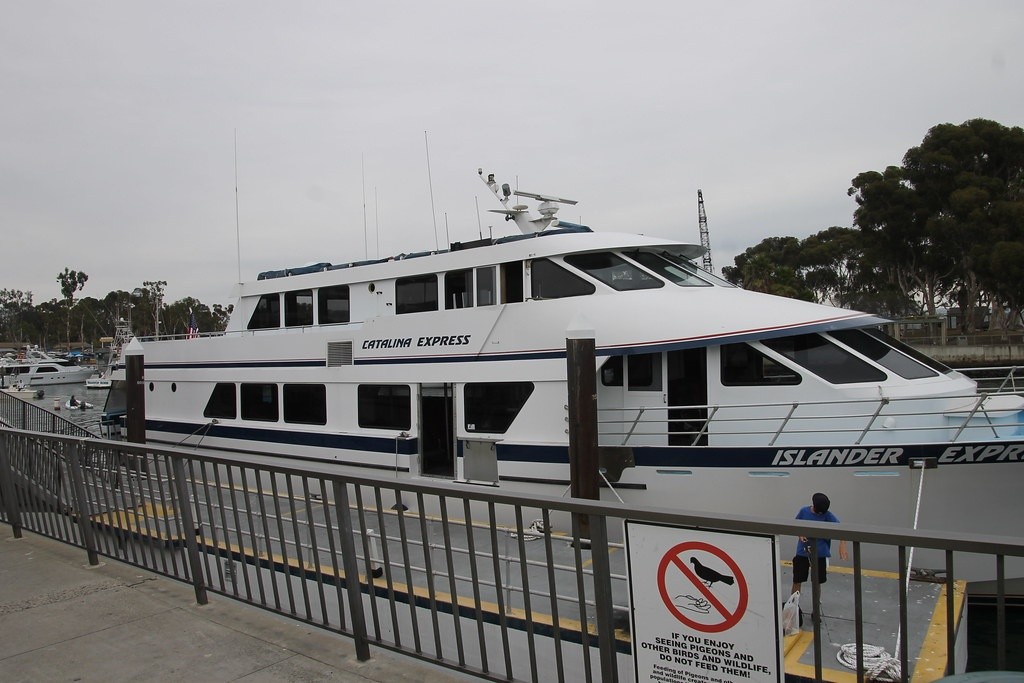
[812,493,830,515]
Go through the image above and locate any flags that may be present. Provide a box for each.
[189,307,200,338]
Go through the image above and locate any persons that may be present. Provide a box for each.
[70,395,79,406]
[790,492,849,624]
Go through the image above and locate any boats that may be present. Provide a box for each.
[0,345,115,410]
[102,166,1023,683]
